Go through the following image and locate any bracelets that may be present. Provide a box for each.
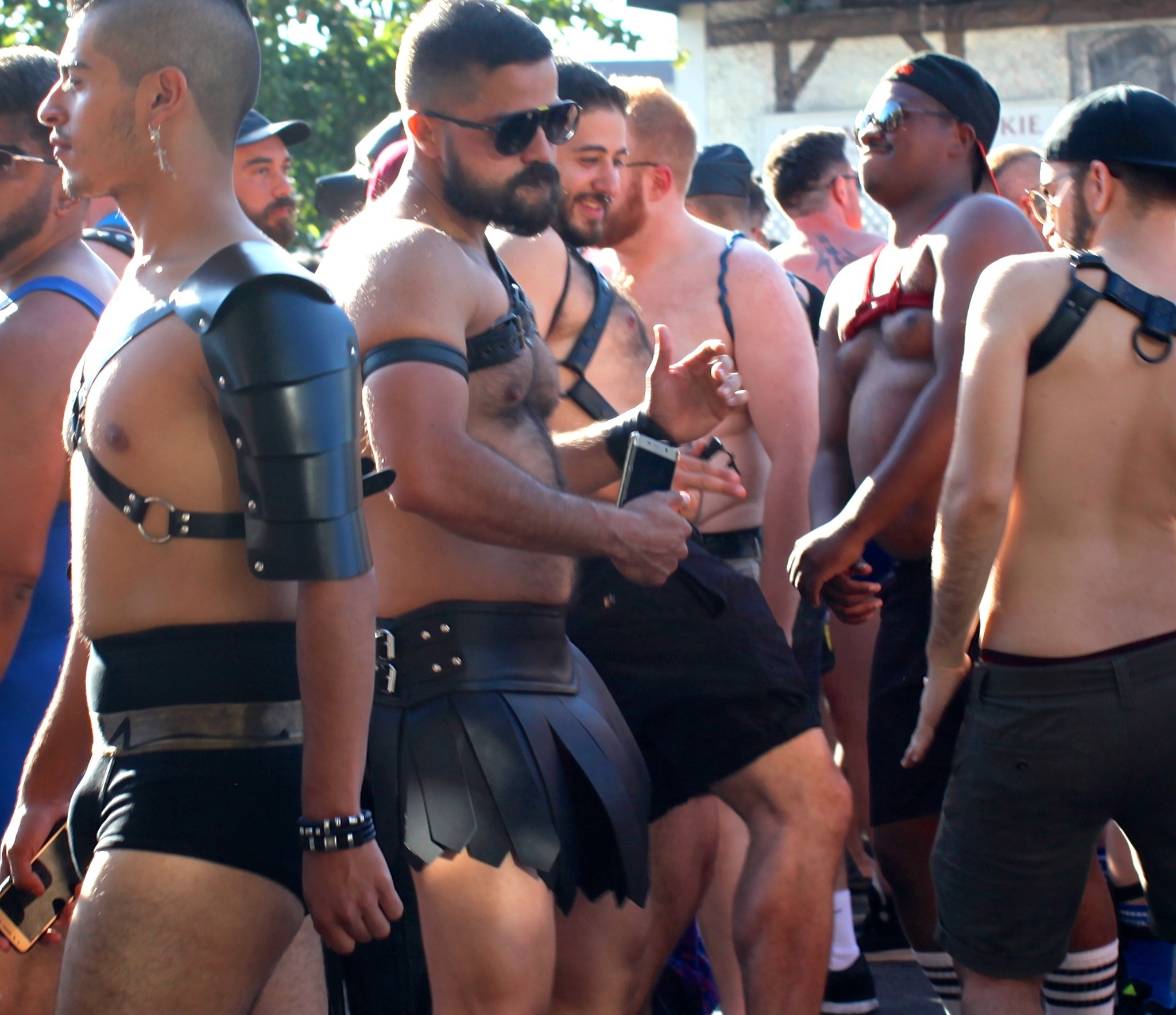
[292,808,377,852]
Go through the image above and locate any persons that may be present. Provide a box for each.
[0,0,1176,1015]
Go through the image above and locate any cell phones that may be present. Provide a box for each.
[617,432,679,507]
[0,823,84,955]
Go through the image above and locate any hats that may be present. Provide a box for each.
[313,110,408,193]
[886,51,1000,197]
[1044,83,1176,169]
[686,143,755,199]
[367,139,411,200]
[235,107,312,146]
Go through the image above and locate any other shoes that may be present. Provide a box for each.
[820,950,878,1015]
[855,911,919,964]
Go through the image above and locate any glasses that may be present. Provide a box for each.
[423,99,583,156]
[1028,169,1121,226]
[853,102,961,148]
[824,172,861,191]
[1,150,62,173]
